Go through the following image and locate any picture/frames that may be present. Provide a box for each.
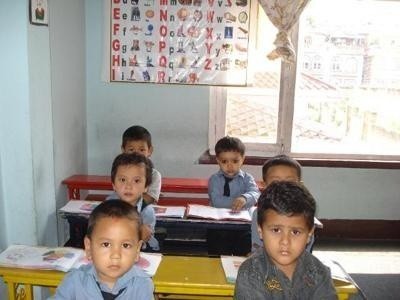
[30,0,48,26]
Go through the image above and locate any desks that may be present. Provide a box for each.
[58,173,324,254]
[1,244,357,299]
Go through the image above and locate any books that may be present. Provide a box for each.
[187,202,252,222]
[219,254,367,299]
[61,196,186,219]
[0,243,164,280]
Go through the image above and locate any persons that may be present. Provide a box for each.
[47,200,155,300]
[207,135,261,256]
[250,153,317,257]
[104,152,162,253]
[119,125,162,206]
[233,180,339,300]
